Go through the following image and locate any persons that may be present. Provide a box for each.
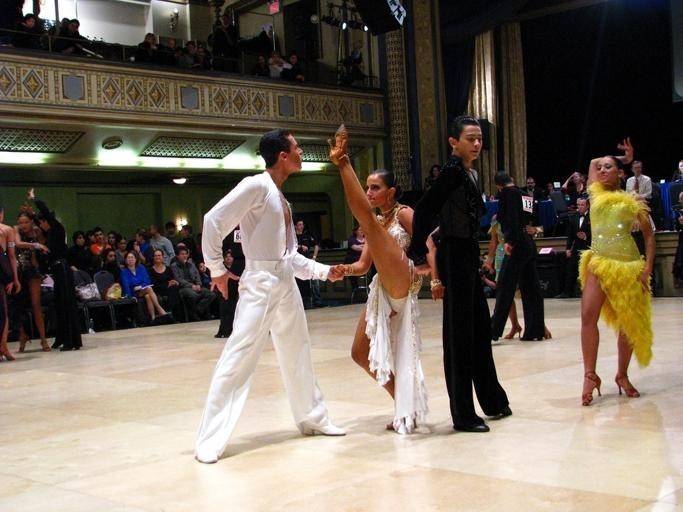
[479,160,683,341]
[327,123,444,435]
[194,129,347,463]
[577,137,656,406]
[12,14,306,81]
[406,115,512,432]
[424,165,440,190]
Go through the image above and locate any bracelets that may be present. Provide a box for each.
[430,279,442,291]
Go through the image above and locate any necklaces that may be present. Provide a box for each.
[381,201,399,215]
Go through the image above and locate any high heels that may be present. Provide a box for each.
[19,335,32,352]
[215,332,231,338]
[520,334,543,341]
[615,373,640,398]
[197,451,218,464]
[303,421,346,436]
[504,326,522,339]
[51,339,80,351]
[0,343,15,361]
[582,372,601,407]
[40,341,50,351]
[386,416,417,431]
[327,123,349,167]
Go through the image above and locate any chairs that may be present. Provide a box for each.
[29,292,190,335]
[350,274,368,305]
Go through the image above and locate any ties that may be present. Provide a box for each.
[635,176,639,194]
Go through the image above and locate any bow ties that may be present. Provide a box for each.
[579,214,586,218]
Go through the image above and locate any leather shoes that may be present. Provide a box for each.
[453,424,491,433]
[489,406,512,416]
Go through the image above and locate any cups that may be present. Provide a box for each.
[660,179,665,184]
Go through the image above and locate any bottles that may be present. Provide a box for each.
[481,193,494,203]
